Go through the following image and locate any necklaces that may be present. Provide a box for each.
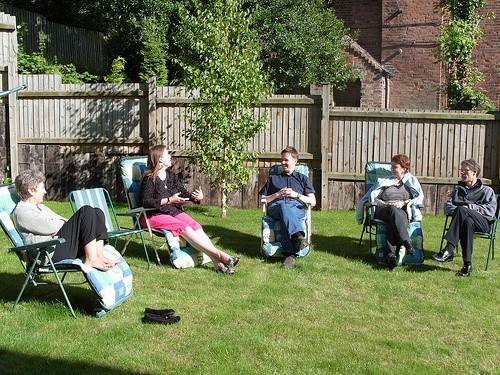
[163,179,167,188]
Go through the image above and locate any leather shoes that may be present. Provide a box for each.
[458,265,472,275]
[431,248,454,262]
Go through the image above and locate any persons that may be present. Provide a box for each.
[138,145,241,274]
[374,153,416,271]
[12,168,120,272]
[258,146,316,269]
[432,158,498,276]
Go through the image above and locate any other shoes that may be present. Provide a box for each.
[145,308,175,315]
[405,243,415,256]
[144,313,180,324]
[294,239,309,252]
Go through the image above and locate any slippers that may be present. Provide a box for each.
[215,266,234,275]
[226,256,239,266]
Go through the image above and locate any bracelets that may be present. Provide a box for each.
[167,197,171,205]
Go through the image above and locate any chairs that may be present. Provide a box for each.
[119,156,213,270]
[358,162,425,269]
[440,194,500,271]
[69,187,150,270]
[0,182,132,319]
[259,164,312,260]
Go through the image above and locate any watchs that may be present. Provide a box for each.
[296,193,301,199]
